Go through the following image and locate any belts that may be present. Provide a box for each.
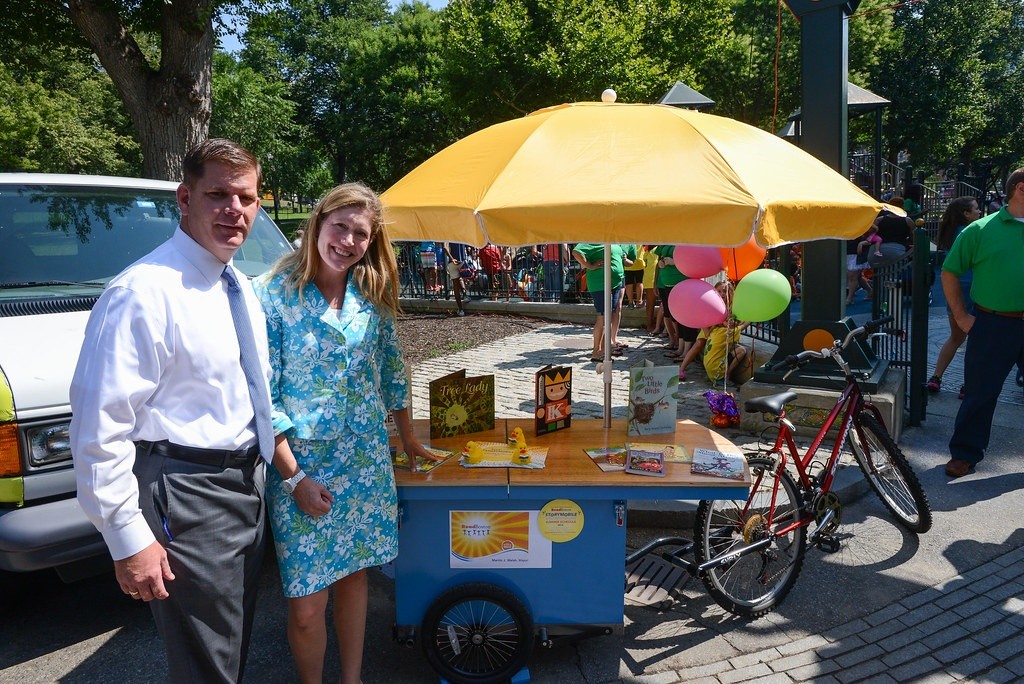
[975,304,1024,319]
[138,438,264,468]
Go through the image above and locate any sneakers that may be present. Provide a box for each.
[927,375,943,391]
[959,384,965,399]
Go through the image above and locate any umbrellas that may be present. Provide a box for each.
[378,89,907,428]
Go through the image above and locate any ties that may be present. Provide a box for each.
[220,265,274,466]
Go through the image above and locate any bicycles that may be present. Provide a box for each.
[661,314,934,618]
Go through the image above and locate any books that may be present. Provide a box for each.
[381,366,413,436]
[627,359,679,436]
[428,368,496,439]
[626,449,665,478]
[394,445,460,473]
[583,446,626,472]
[534,363,572,436]
[624,442,692,463]
[691,447,745,481]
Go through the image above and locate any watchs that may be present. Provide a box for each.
[280,470,306,494]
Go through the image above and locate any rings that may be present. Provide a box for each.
[129,591,138,595]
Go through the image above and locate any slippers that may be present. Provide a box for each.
[715,379,735,387]
[603,350,623,357]
[646,327,686,362]
[617,342,628,348]
[590,355,614,363]
[611,344,623,351]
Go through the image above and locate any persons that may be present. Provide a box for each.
[847,173,935,305]
[927,197,981,399]
[988,191,1007,215]
[941,165,1024,477]
[68,137,275,684]
[251,183,438,684]
[420,241,751,386]
[292,230,304,249]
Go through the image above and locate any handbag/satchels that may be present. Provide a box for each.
[460,260,476,279]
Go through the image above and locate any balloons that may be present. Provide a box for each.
[725,234,767,281]
[803,329,835,352]
[673,245,723,279]
[732,268,792,322]
[668,279,727,329]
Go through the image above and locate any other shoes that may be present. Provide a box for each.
[864,293,872,300]
[457,310,465,316]
[464,296,472,303]
[679,370,687,381]
[628,303,635,310]
[944,458,970,477]
[846,300,854,305]
[636,300,646,308]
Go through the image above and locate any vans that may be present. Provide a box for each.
[0,171,298,575]
